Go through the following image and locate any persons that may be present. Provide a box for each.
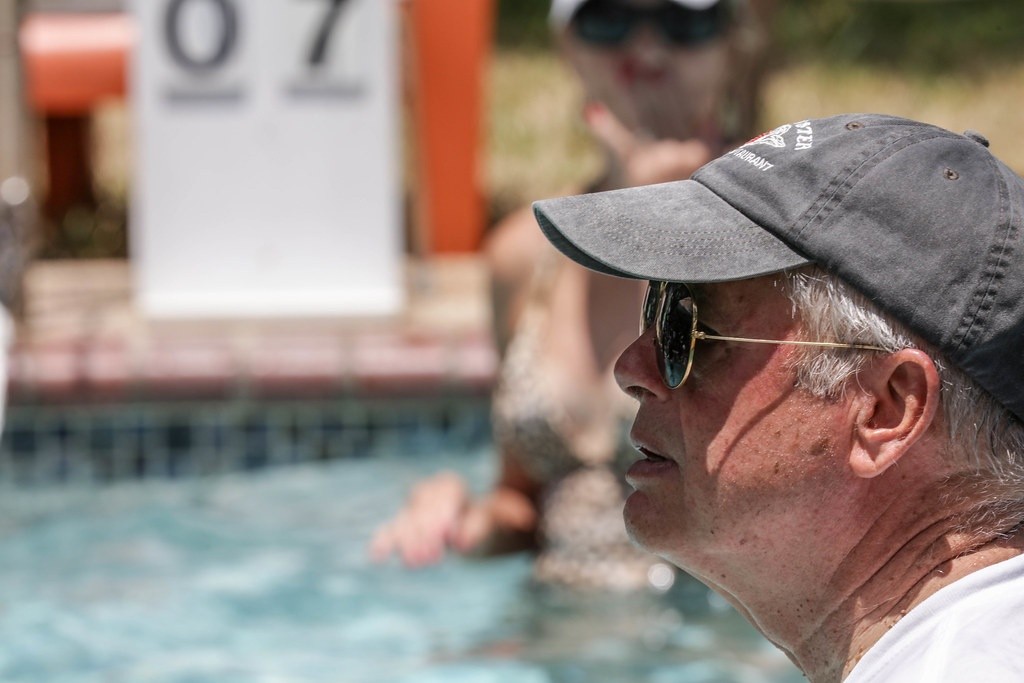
[530,112,1021,683]
[367,0,773,598]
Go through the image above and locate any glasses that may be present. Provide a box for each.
[572,0,735,49]
[639,280,891,390]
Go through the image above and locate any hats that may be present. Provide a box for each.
[532,112,1024,421]
